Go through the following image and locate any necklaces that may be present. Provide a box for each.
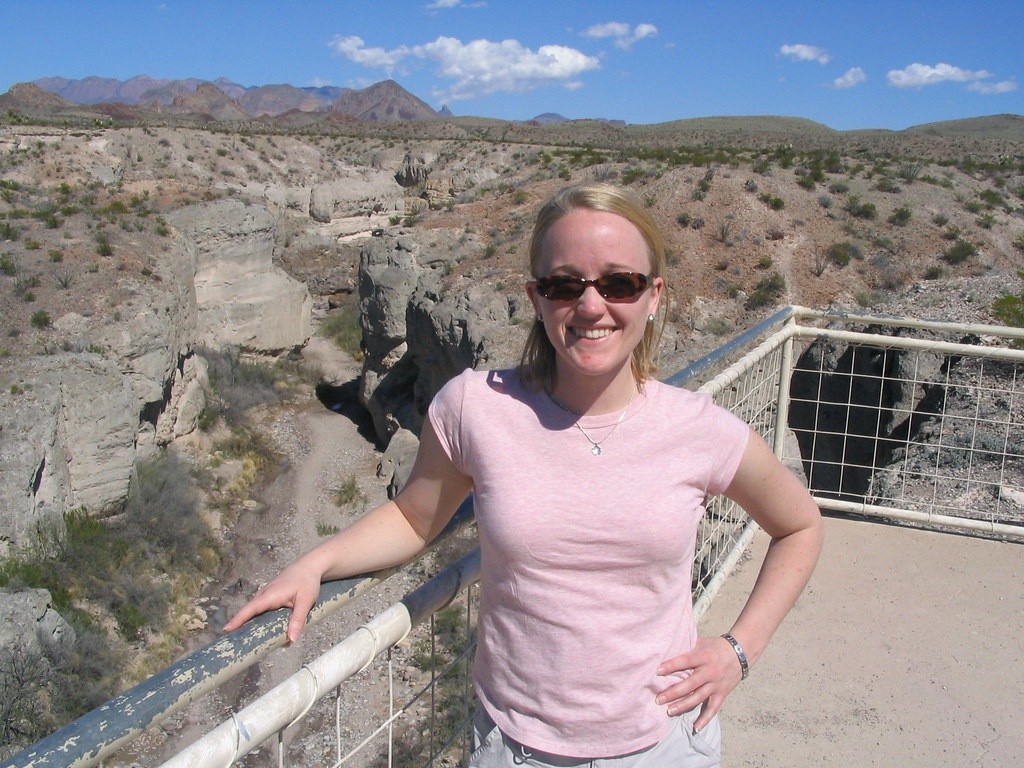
[552,377,635,457]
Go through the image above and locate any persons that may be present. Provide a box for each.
[223,182,825,768]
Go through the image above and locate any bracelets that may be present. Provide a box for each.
[721,634,749,680]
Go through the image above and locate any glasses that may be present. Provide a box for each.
[535,271,654,303]
